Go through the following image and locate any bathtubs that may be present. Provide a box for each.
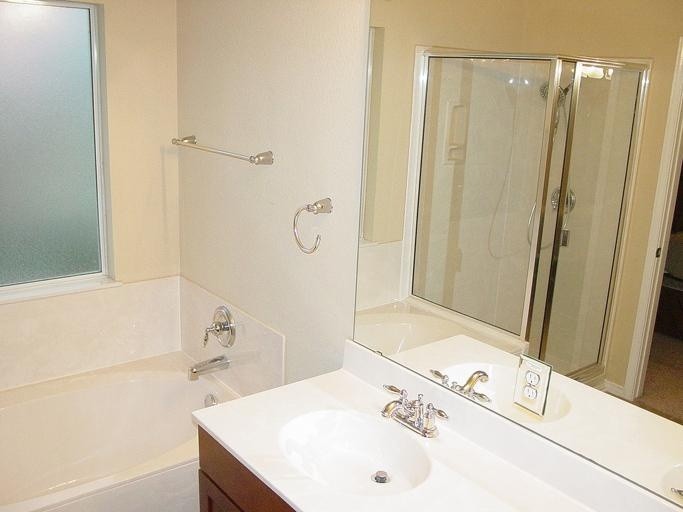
[0,351,240,512]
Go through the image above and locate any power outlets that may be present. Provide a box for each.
[514,353,552,416]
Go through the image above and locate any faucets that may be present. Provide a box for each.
[381,384,449,438]
[185,354,230,382]
[430,368,493,402]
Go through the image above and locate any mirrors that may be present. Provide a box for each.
[352,0,683,512]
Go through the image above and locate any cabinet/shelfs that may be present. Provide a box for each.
[198,427,294,512]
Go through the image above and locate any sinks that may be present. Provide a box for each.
[441,354,573,424]
[274,405,434,502]
